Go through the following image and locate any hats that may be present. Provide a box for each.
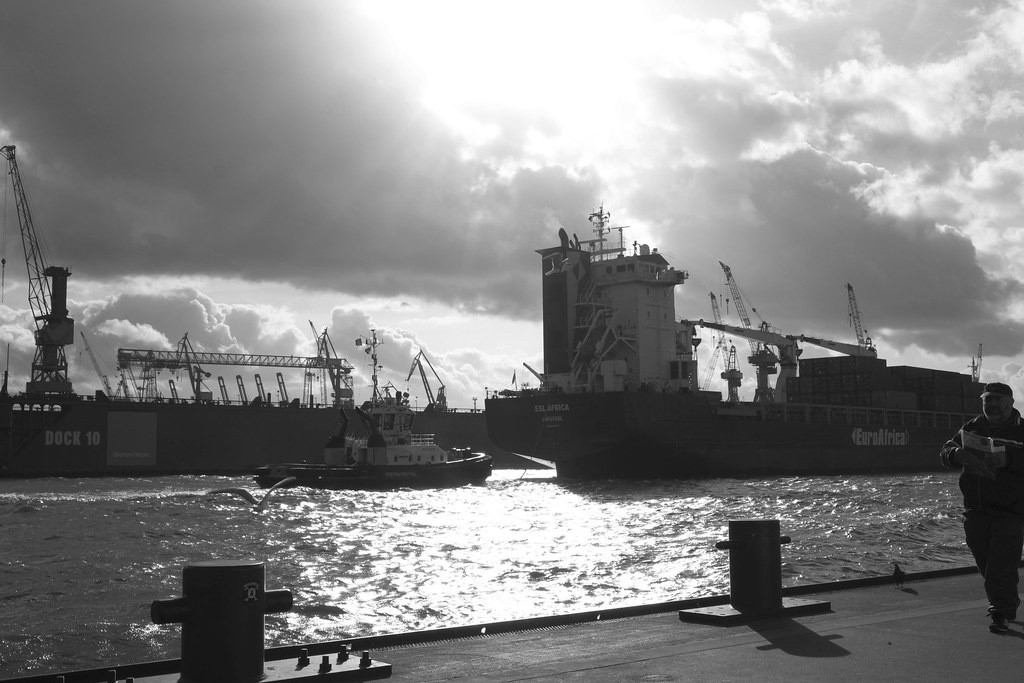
[979,383,1012,398]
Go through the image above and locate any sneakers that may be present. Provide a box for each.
[986,609,1009,632]
[1007,611,1016,620]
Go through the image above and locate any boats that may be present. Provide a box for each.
[252,325,495,493]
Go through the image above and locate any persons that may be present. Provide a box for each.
[937,381,1024,634]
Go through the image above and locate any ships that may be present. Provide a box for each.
[485,199,991,479]
[0,146,485,472]
